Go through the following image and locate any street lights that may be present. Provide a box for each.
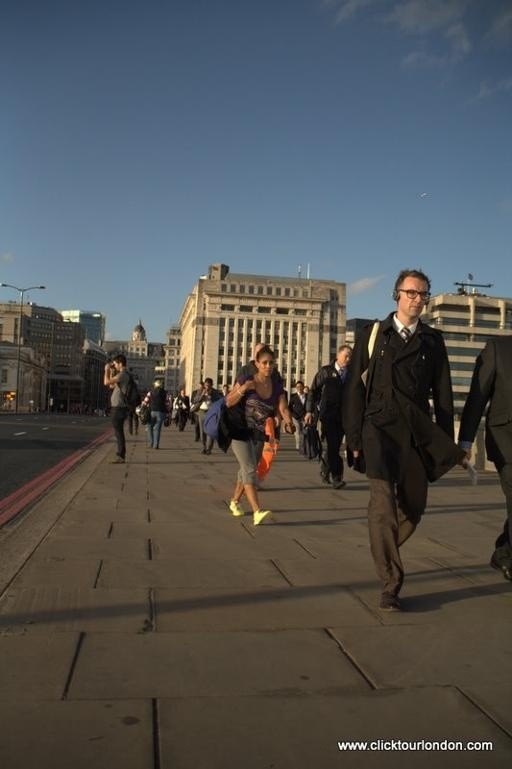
[35,315,71,415]
[0,283,45,415]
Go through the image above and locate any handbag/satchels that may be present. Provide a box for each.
[202,397,226,439]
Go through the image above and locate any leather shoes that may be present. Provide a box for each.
[378,590,402,612]
[490,552,512,582]
[319,470,332,484]
[332,480,346,489]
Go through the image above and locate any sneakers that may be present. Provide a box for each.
[252,509,272,526]
[230,497,245,517]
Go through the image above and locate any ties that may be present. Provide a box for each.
[400,327,412,346]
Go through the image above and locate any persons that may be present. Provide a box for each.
[62,344,350,528]
[343,270,467,613]
[457,335,510,580]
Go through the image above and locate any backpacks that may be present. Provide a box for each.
[300,423,322,461]
[117,370,142,413]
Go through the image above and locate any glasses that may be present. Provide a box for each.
[396,288,431,301]
